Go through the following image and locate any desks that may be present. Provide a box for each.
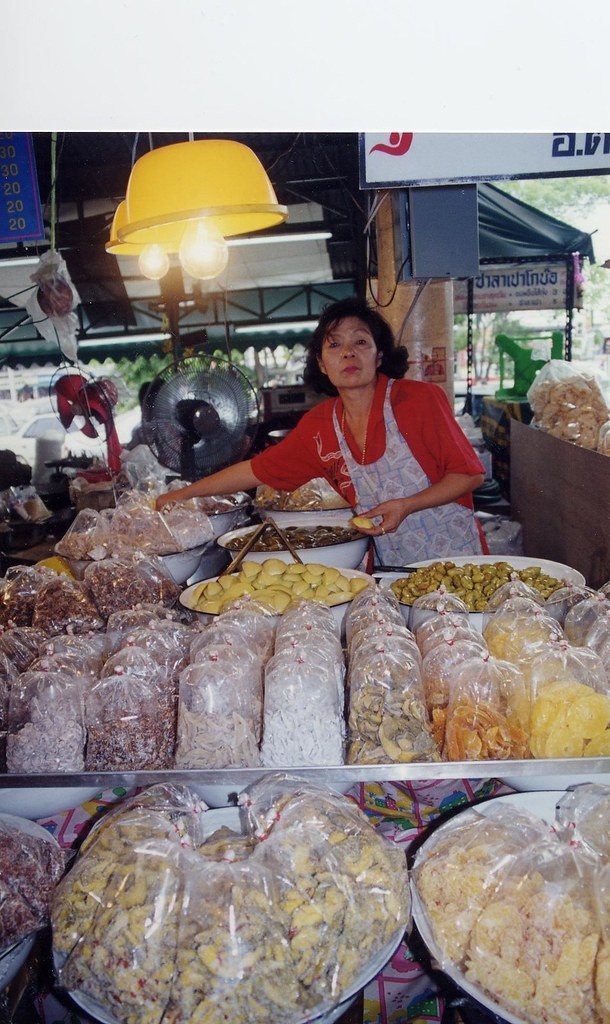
[480,396,534,486]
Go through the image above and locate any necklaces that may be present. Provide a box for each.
[342,404,369,504]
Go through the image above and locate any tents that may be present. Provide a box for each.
[0,182,593,400]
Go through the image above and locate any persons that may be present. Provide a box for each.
[133,379,184,472]
[155,296,490,584]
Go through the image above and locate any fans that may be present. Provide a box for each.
[140,354,262,478]
[46,366,121,482]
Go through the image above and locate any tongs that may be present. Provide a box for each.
[216,515,306,582]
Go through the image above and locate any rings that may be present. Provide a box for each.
[380,526,386,535]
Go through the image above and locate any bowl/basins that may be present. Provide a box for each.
[178,567,376,638]
[166,487,249,546]
[53,532,213,585]
[408,789,610,1024]
[0,812,64,994]
[216,517,370,569]
[51,807,413,1024]
[250,500,354,521]
[379,554,586,635]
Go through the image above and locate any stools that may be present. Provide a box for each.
[495,332,563,402]
